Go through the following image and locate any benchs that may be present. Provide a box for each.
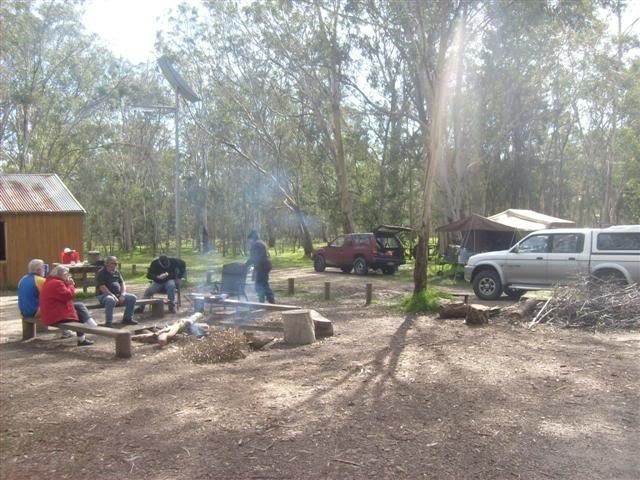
[36,298,164,332]
[194,296,300,316]
[21,316,131,358]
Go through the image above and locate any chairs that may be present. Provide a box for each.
[154,277,182,311]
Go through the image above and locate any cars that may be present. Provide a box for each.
[463,223,639,303]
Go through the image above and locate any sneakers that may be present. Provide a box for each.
[61,330,76,338]
[123,318,138,325]
[78,338,94,345]
[105,322,111,326]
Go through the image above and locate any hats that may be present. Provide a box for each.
[159,255,169,266]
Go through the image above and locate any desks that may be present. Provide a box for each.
[53,262,102,295]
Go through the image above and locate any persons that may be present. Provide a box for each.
[18,259,74,338]
[39,265,98,345]
[134,254,187,313]
[95,256,138,328]
[242,229,275,304]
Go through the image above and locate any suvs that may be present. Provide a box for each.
[309,224,413,277]
